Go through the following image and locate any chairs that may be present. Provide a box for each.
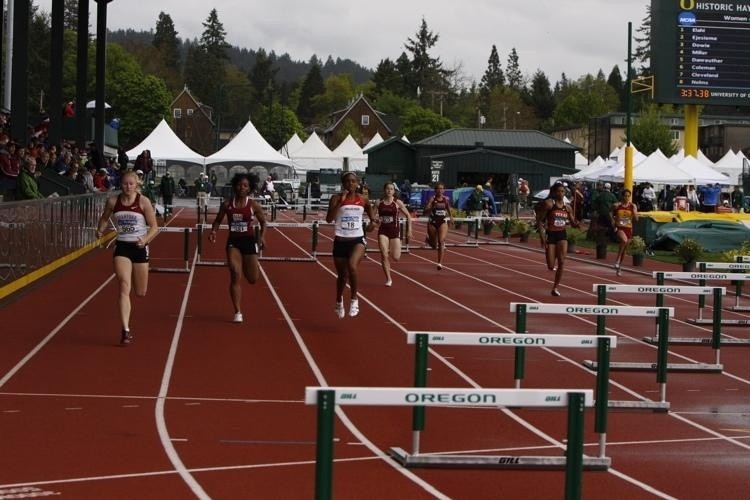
[174,181,236,199]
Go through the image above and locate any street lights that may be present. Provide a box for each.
[476,107,487,128]
[513,110,521,129]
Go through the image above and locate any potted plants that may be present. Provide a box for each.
[726,246,749,283]
[448,188,617,256]
[628,236,646,266]
[673,238,703,273]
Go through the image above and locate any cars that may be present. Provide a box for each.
[265,178,433,216]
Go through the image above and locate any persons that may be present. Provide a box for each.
[326,172,380,320]
[563,181,745,228]
[261,176,275,197]
[516,178,529,208]
[95,171,160,345]
[536,183,580,295]
[608,189,638,277]
[0,110,220,218]
[466,177,494,236]
[361,176,413,287]
[423,182,453,269]
[209,171,268,323]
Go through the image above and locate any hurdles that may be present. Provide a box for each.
[508,302,674,415]
[303,386,594,500]
[389,332,617,468]
[581,255,750,373]
[145,193,513,272]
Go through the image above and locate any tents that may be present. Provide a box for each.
[578,142,750,206]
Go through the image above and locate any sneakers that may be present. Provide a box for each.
[334,295,345,320]
[348,298,359,317]
[232,311,243,322]
[120,330,134,345]
